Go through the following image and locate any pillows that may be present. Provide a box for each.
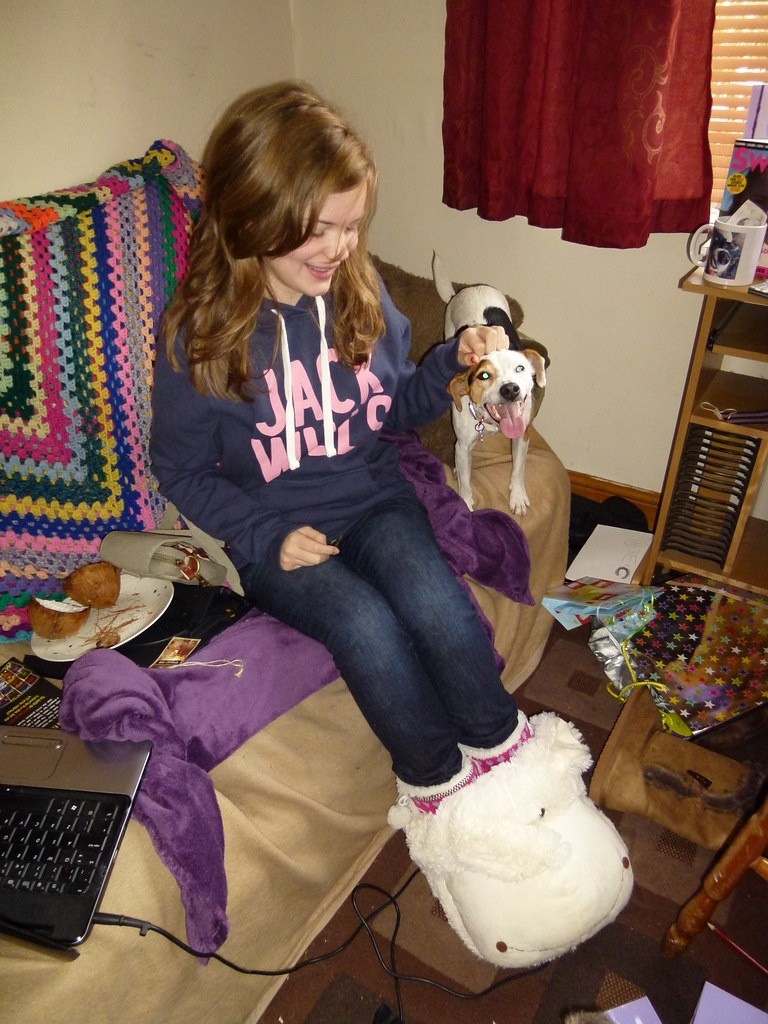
[369,255,524,469]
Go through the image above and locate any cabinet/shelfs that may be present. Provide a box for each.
[642,264,768,609]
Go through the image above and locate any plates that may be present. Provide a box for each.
[30,573,173,662]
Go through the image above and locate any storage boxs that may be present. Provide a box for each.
[719,137,768,278]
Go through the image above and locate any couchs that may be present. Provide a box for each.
[0,137,571,1024]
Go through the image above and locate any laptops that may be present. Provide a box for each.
[1,721,154,954]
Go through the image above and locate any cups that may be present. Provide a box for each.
[687,216,768,286]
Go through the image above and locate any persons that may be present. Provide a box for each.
[153,82,635,967]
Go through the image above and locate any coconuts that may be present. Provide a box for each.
[61,561,121,609]
[27,594,91,639]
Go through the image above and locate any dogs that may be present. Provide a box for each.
[431,249,546,517]
